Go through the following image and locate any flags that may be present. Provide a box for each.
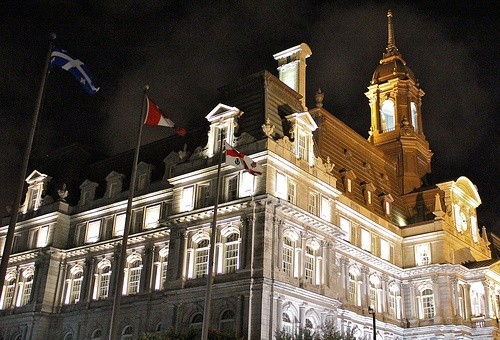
[47,45,100,94]
[225,141,262,178]
[143,94,185,136]
